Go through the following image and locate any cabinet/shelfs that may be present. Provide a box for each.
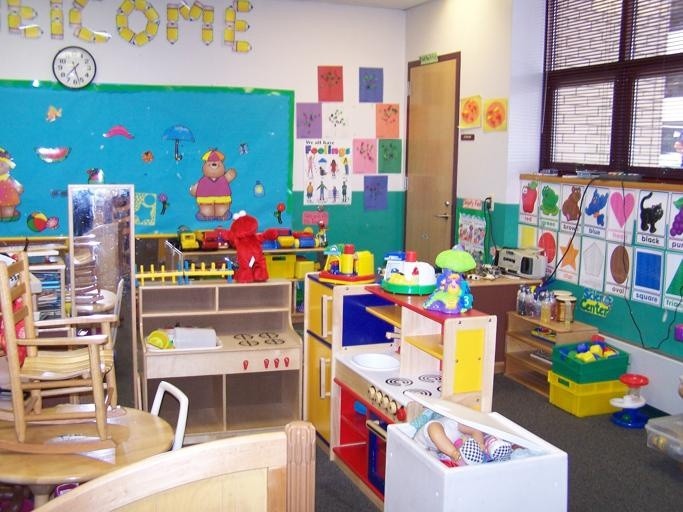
[327,283,495,512]
[500,311,598,400]
[138,278,306,446]
[302,272,379,459]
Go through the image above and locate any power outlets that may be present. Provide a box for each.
[485,195,495,212]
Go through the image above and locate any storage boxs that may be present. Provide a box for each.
[643,412,682,460]
[550,340,630,384]
[546,370,629,417]
[265,254,295,279]
[380,406,572,511]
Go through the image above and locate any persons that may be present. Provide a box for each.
[402,399,544,468]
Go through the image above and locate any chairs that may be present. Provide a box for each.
[27,420,319,512]
[73,235,103,305]
[81,279,124,380]
[0,247,120,439]
[150,378,189,449]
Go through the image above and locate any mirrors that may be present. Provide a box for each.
[67,184,138,413]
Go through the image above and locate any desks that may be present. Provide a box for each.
[163,239,329,326]
[0,405,173,509]
[76,288,116,335]
[468,275,544,379]
[26,254,65,335]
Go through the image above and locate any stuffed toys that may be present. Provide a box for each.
[216,210,279,284]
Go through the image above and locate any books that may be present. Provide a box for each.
[0,237,104,337]
[0,349,32,424]
[528,348,552,366]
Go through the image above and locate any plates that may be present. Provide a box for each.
[379,280,434,296]
[316,271,377,286]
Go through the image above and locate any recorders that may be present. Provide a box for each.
[495,247,549,278]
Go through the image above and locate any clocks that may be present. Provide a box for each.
[50,46,96,90]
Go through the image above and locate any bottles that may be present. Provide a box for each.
[515,283,575,328]
[390,250,419,287]
[330,244,355,275]
